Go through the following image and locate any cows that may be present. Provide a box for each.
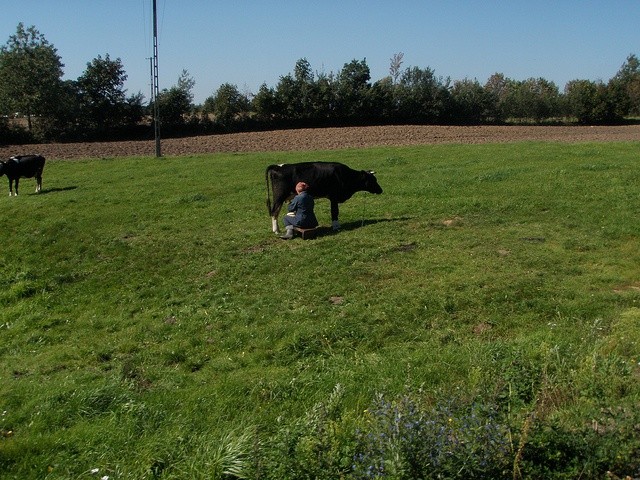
[266,162,383,235]
[0,154,45,196]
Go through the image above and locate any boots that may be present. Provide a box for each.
[279,225,294,240]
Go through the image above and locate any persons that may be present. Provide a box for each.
[280,182,318,239]
